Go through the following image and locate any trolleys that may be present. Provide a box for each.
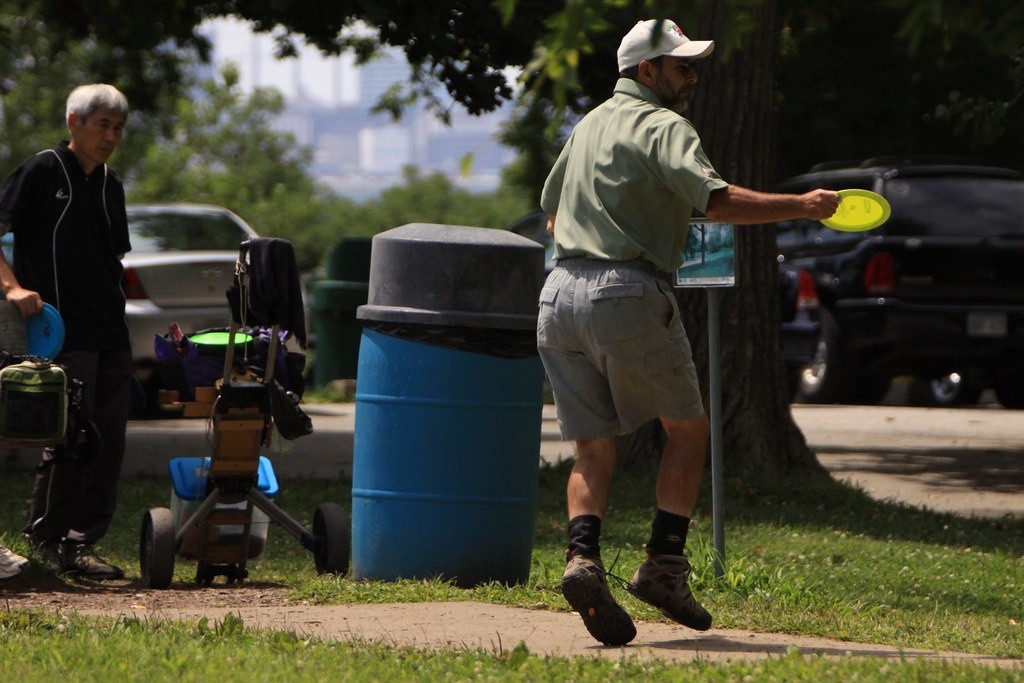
[138,234,351,587]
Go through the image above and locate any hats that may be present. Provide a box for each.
[617,19,715,72]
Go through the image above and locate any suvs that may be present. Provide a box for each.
[764,154,1023,410]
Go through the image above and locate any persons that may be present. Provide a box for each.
[1,84,132,578]
[536,17,842,647]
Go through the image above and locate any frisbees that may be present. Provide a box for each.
[818,189,892,233]
[26,301,65,360]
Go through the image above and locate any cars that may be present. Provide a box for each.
[119,201,312,369]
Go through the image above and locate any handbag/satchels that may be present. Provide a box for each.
[226,237,306,351]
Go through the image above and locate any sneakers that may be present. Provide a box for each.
[58,541,123,580]
[0,544,28,578]
[562,557,637,646]
[629,548,712,630]
[28,538,64,573]
